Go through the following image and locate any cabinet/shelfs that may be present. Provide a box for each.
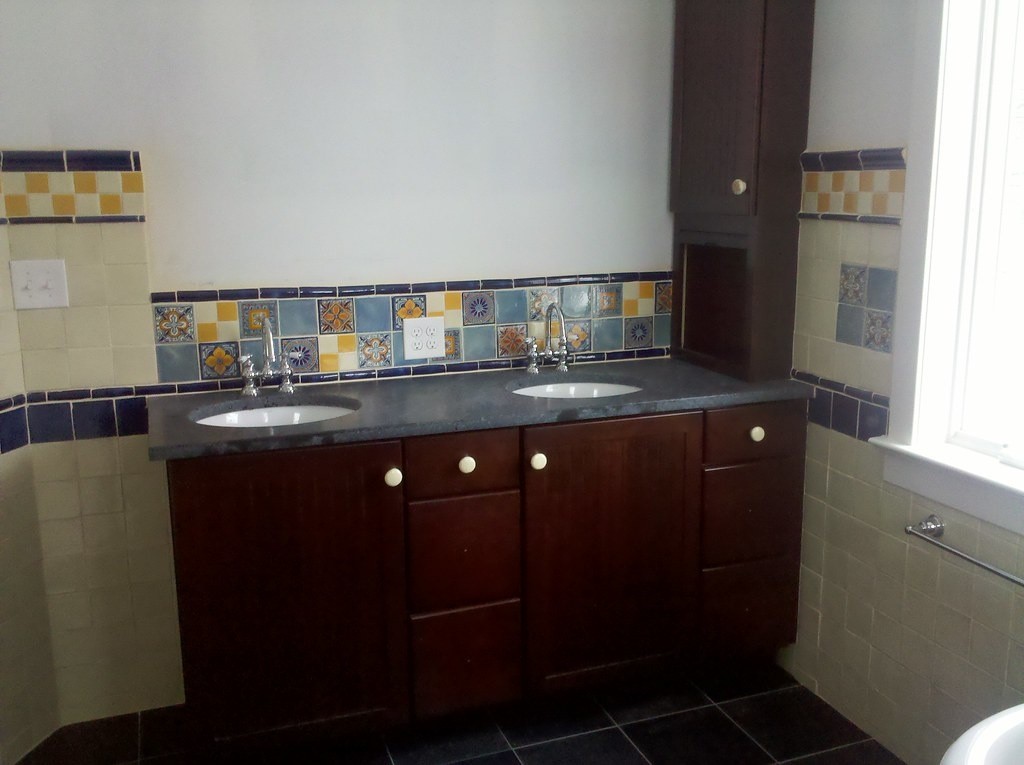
[165,428,524,765]
[521,399,809,705]
[668,0,817,382]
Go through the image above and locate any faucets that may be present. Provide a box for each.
[234,315,297,396]
[521,301,577,376]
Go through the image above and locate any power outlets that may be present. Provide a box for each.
[403,316,445,360]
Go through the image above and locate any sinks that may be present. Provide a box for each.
[503,367,648,400]
[184,391,363,428]
